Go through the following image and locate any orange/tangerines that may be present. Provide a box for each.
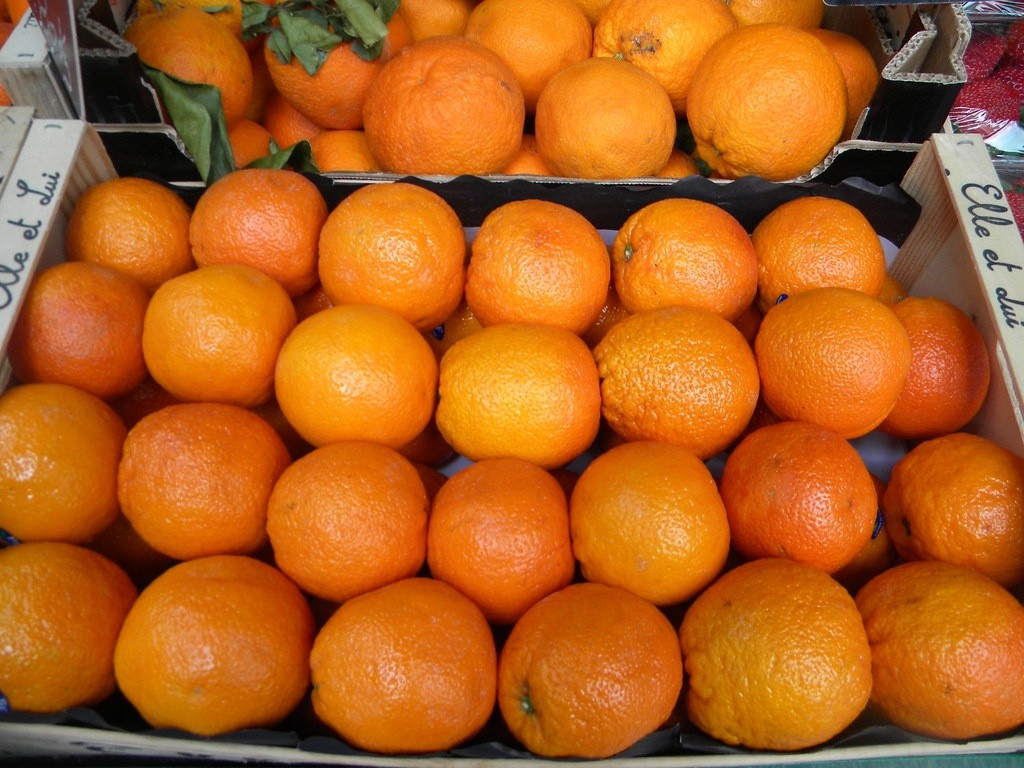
[121,0,881,185]
[0,191,1023,753]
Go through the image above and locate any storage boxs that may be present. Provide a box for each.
[0,0,1024,768]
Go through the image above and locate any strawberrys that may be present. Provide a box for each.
[945,18,1024,240]
